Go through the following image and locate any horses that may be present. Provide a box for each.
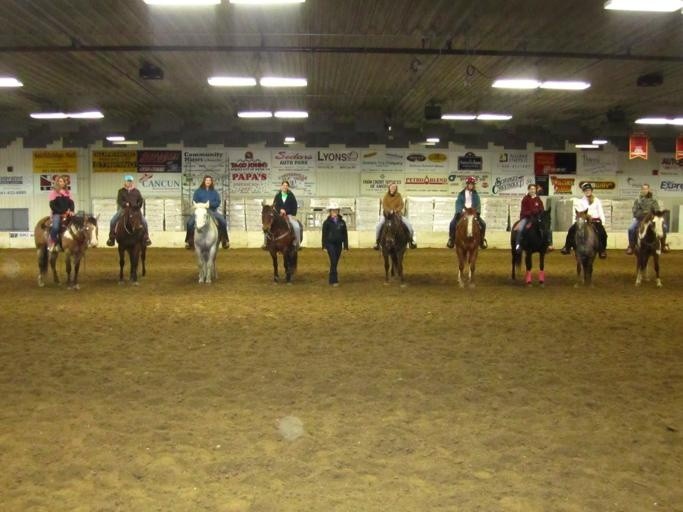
[454,206,482,289]
[192,200,221,284]
[378,207,408,287]
[34,213,99,291]
[262,201,303,286]
[572,208,599,288]
[635,206,667,288]
[511,206,551,289]
[113,202,147,287]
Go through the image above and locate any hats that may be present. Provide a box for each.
[326,202,341,209]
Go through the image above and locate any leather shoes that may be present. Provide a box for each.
[144,239,151,246]
[185,241,190,249]
[626,247,633,254]
[561,247,570,254]
[600,251,607,258]
[222,241,228,249]
[107,239,112,245]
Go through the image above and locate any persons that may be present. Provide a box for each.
[446,176,488,249]
[261,179,302,251]
[372,182,417,251]
[62,175,70,185]
[52,175,59,185]
[106,175,152,247]
[561,184,607,259]
[626,182,671,255]
[514,184,558,251]
[322,203,353,288]
[46,175,75,253]
[183,174,230,250]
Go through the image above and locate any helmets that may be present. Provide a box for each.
[123,175,133,181]
[582,184,592,191]
[465,176,476,184]
